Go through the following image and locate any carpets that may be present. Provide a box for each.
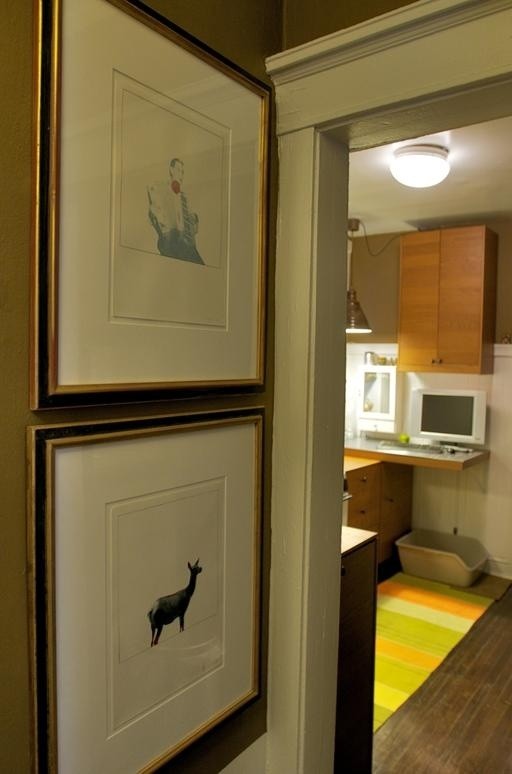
[372,571,495,736]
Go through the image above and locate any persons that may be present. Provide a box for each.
[143,153,208,266]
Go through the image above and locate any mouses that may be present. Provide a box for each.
[447,447,454,456]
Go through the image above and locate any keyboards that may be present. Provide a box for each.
[381,440,443,457]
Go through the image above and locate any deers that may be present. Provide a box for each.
[148,557,203,647]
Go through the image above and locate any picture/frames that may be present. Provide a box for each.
[31,0,271,411]
[26,405,264,774]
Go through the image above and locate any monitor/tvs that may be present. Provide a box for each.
[409,387,488,454]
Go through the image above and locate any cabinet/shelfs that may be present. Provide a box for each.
[346,462,413,562]
[397,225,497,374]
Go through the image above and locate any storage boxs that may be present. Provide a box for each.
[395,529,488,588]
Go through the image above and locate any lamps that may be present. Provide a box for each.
[345,219,373,335]
[389,143,450,189]
